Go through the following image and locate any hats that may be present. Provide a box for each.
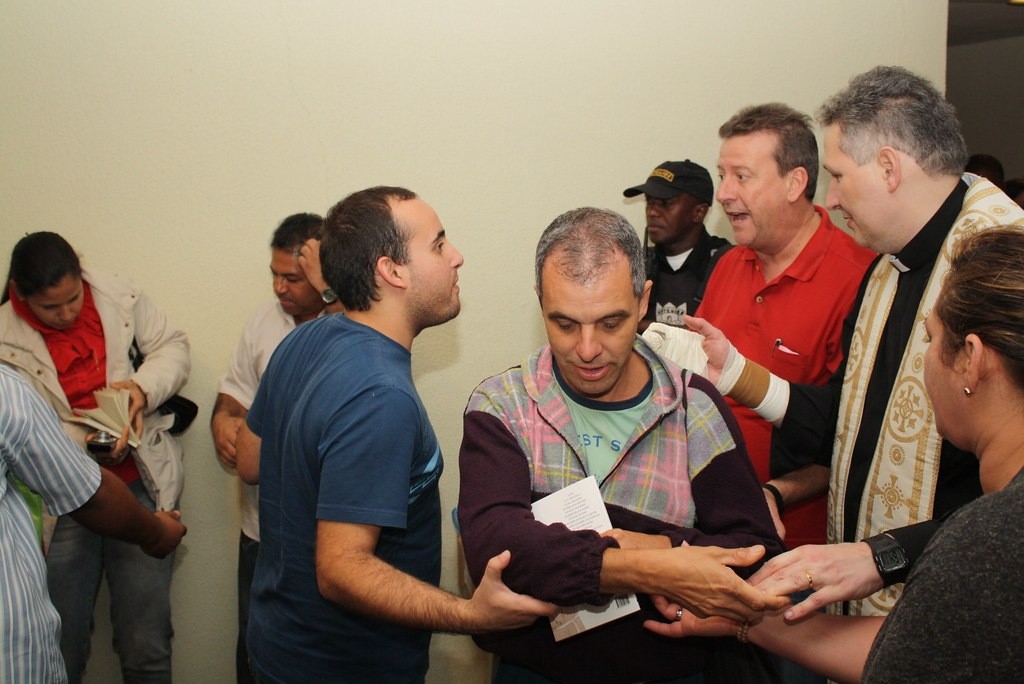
[623,159,713,206]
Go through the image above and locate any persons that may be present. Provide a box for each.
[210,212,342,684]
[0,231,190,683]
[458,207,801,684]
[623,160,731,327]
[691,103,877,684]
[0,366,187,684]
[965,154,1024,209]
[641,66,1024,620]
[643,227,1024,684]
[237,185,560,683]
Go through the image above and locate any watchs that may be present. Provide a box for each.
[860,533,908,588]
[322,288,338,304]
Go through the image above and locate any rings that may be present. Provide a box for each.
[677,609,682,618]
[806,572,813,587]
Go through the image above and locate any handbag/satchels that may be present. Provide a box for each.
[128,336,199,435]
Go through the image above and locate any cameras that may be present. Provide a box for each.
[87,431,118,453]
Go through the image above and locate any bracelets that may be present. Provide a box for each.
[762,483,785,516]
[737,624,748,642]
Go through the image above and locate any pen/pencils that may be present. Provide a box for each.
[771,338,783,359]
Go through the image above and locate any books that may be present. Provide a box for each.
[66,386,142,447]
[533,476,641,643]
